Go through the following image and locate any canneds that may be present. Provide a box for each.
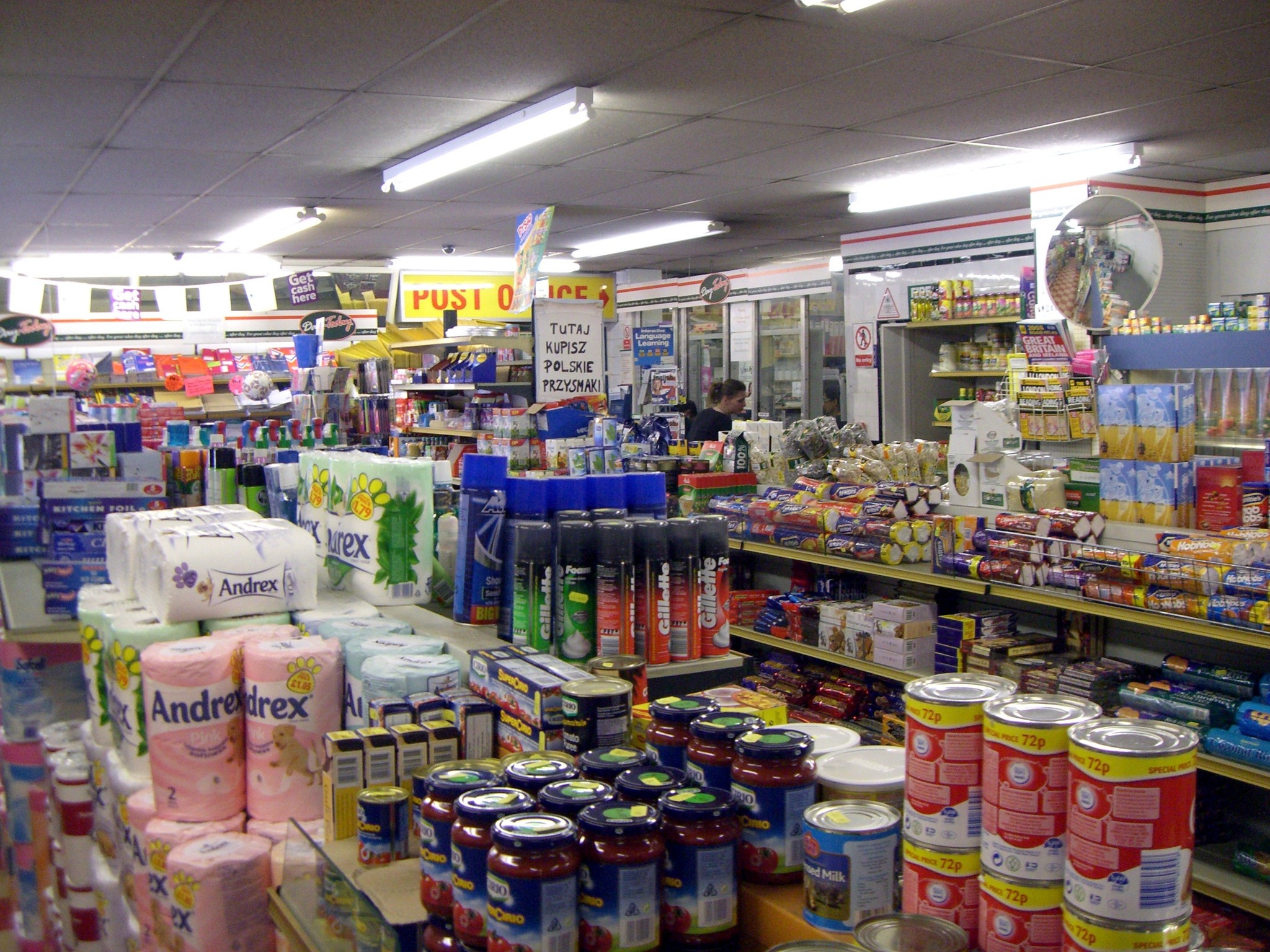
[356,655,816,952]
[955,291,1021,318]
[938,279,974,320]
[799,668,1198,952]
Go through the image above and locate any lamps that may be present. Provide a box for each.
[13,89,731,276]
[848,141,1144,213]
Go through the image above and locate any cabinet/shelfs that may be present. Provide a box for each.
[388,334,534,438]
[725,540,994,684]
[990,580,1270,926]
[1,376,293,421]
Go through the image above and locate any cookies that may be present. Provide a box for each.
[1116,655,1187,722]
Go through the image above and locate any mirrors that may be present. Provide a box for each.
[1044,194,1163,330]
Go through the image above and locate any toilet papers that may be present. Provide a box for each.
[102,503,320,629]
[0,576,460,952]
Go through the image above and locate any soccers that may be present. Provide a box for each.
[243,370,274,401]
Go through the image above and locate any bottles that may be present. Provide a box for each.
[433,452,731,667]
[165,448,299,525]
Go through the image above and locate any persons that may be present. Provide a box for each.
[686,379,746,442]
[657,400,698,438]
[651,377,671,403]
[823,385,847,430]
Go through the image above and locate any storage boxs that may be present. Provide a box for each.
[322,645,597,837]
[818,596,1055,673]
[0,421,169,616]
[1066,382,1270,531]
[939,399,1023,510]
[427,348,593,470]
